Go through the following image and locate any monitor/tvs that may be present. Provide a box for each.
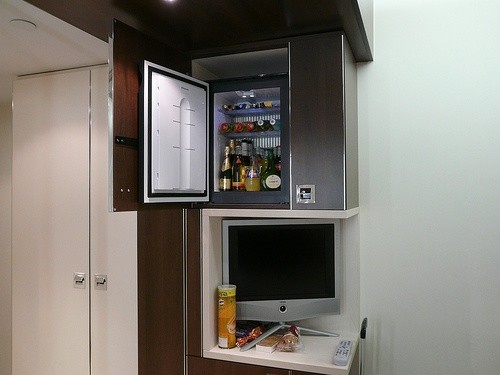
[221,219,341,352]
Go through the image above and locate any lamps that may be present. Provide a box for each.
[7,19,38,32]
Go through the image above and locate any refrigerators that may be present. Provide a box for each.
[108,17,359,212]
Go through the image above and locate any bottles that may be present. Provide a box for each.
[218,138,281,192]
[222,101,280,111]
[219,119,280,133]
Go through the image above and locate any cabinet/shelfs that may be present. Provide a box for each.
[12,63,138,375]
[113,0,374,374]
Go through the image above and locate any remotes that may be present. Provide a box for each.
[334,339,353,366]
